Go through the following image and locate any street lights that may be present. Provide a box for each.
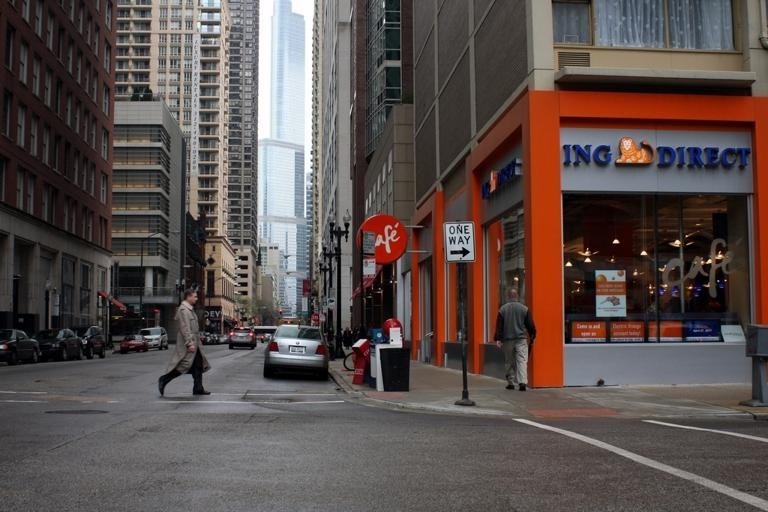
[44,278,53,330]
[174,278,185,307]
[287,285,302,290]
[285,270,305,275]
[281,252,308,260]
[207,276,225,334]
[138,230,180,329]
[317,206,352,360]
[239,307,246,326]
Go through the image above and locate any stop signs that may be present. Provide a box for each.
[311,312,319,321]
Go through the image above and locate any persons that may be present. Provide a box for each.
[342,322,362,350]
[495,288,537,391]
[158,289,211,396]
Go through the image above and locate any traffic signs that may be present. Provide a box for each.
[442,221,475,263]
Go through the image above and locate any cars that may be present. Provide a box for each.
[260,323,329,383]
[118,333,151,355]
[0,325,108,370]
[197,325,278,352]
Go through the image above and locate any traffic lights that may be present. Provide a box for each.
[279,309,282,315]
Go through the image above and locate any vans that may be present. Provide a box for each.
[135,326,170,352]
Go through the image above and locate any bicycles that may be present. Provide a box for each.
[342,350,355,371]
